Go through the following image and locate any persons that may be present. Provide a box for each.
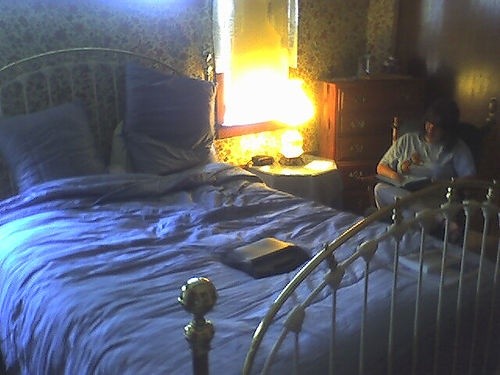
[374,98,489,247]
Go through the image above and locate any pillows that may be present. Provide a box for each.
[123,68,217,180]
[1,100,108,196]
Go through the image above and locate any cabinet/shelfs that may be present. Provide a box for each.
[319,75,430,211]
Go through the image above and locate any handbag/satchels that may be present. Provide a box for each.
[224,236,307,280]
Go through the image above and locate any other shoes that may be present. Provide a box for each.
[447,222,463,240]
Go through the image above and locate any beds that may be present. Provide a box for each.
[0,46,500,375]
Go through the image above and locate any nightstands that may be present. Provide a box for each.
[248,158,341,207]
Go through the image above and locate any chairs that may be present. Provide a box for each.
[393,116,485,248]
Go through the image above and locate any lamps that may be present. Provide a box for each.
[260,79,317,170]
